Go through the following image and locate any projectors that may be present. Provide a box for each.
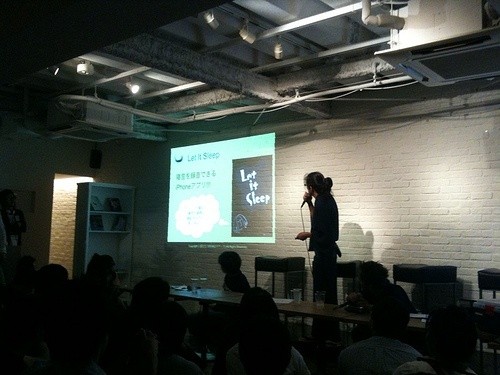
[58,101,134,143]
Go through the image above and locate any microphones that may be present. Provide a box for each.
[300,193,313,207]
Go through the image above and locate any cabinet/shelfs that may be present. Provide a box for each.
[70,182,138,299]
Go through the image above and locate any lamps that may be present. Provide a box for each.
[45,1,407,94]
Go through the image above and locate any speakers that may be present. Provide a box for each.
[90,149,103,169]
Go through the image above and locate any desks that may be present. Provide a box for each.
[169,256,500,375]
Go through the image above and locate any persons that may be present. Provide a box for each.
[0,252,309,375]
[297,172,341,305]
[0,189,27,256]
[337,261,500,375]
[219,251,250,293]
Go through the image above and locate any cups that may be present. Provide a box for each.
[191,278,199,293]
[315,291,325,308]
[293,288,302,304]
[200,278,208,291]
